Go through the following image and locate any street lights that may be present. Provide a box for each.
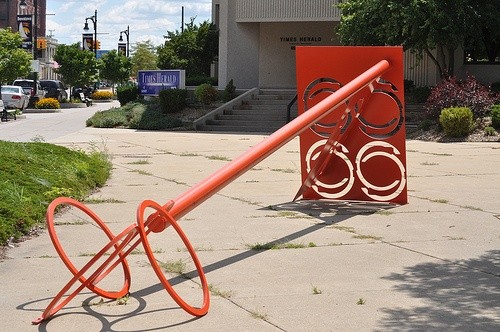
[118,25,129,57]
[18,0,38,95]
[83,9,97,89]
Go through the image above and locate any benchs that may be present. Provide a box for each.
[79,92,92,107]
[0,100,17,121]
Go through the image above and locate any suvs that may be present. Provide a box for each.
[11,80,68,106]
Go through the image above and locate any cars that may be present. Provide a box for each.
[1,85,30,111]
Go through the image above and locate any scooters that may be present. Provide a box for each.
[86,96,93,107]
